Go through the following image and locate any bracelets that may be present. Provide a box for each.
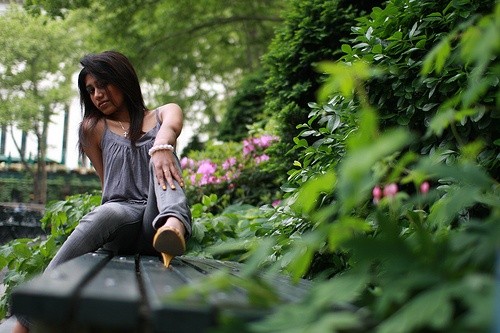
[148,144,174,154]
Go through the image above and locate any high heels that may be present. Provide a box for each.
[153,227,186,269]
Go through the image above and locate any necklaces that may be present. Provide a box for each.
[115,111,132,138]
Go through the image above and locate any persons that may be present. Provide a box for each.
[0,51,193,333]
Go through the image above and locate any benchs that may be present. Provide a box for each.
[8,251,315,333]
